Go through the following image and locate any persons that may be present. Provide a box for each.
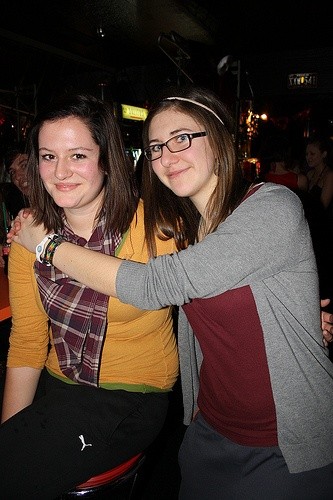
[0,86,333,500]
[302,133,333,292]
[0,142,33,276]
[262,137,309,192]
[7,88,333,500]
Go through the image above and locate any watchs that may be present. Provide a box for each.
[35,233,59,263]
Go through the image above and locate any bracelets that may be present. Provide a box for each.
[43,236,63,265]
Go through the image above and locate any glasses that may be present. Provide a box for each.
[142,131,210,161]
